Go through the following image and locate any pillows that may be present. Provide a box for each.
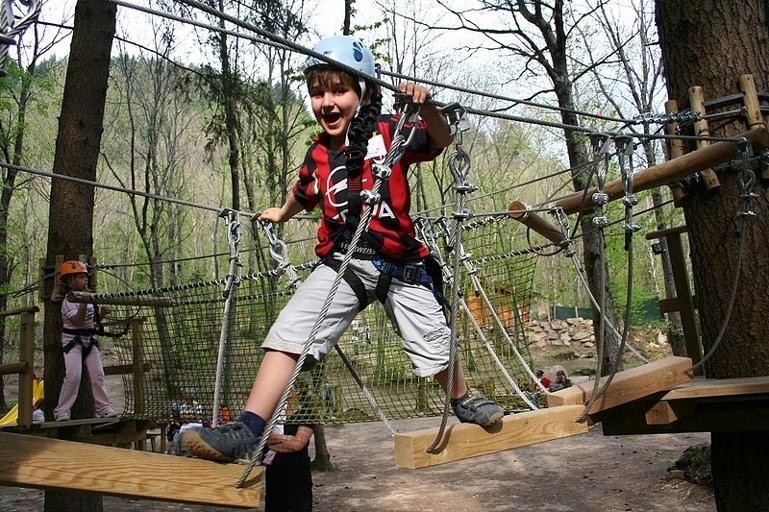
[303,36,376,78]
[59,260,88,280]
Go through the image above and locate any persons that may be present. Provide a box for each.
[173,389,202,454]
[550,369,572,390]
[260,377,317,511]
[537,369,551,387]
[33,398,46,422]
[180,32,505,462]
[52,259,119,421]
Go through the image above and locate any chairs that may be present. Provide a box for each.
[56,412,70,422]
[451,392,505,428]
[95,410,118,417]
[180,421,263,467]
